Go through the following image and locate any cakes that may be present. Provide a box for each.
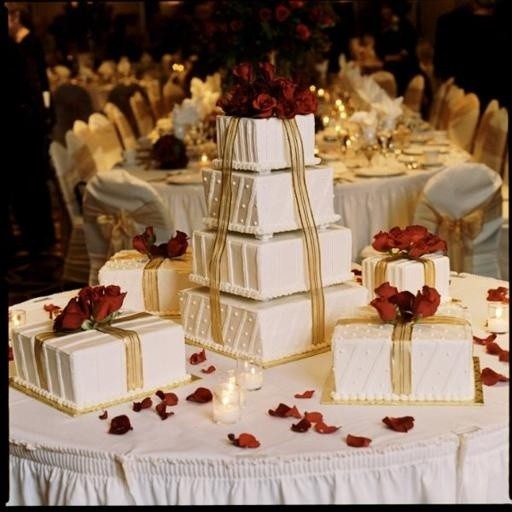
[11,59,476,409]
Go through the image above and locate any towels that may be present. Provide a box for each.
[347,92,404,143]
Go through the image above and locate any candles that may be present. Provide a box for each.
[487,307,506,334]
[212,366,262,424]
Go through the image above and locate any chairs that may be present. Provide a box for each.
[47,82,185,287]
[370,69,512,278]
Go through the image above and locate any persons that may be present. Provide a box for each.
[46,0,91,76]
[4,1,58,253]
[373,0,422,97]
[446,0,512,118]
[92,10,150,71]
[432,0,476,84]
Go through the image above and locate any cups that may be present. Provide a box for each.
[235,354,264,392]
[488,302,510,333]
[210,382,243,425]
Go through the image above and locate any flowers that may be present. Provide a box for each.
[131,226,192,259]
[51,284,128,336]
[213,1,340,121]
[367,223,449,324]
